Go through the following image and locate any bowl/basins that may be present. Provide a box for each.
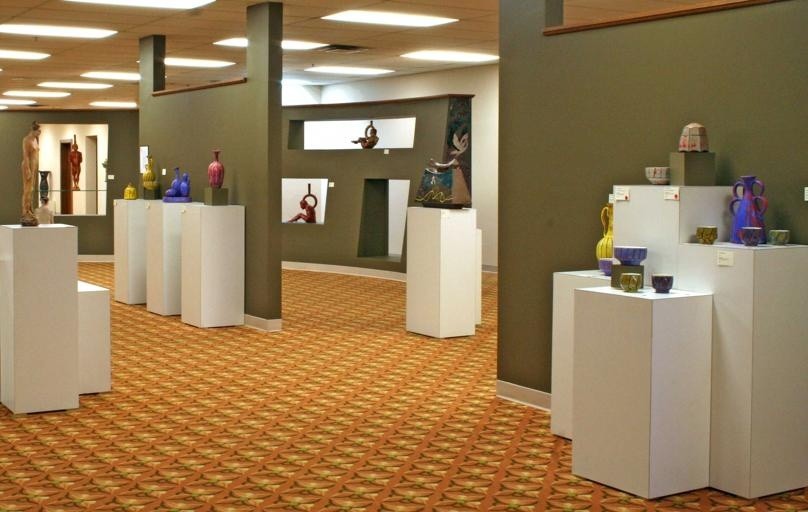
[769,230,791,245]
[736,226,762,245]
[614,245,647,266]
[644,166,671,184]
[620,273,642,292]
[599,257,612,275]
[695,226,718,243]
[652,274,672,292]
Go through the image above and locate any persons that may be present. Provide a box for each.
[352,128,379,149]
[22,120,41,216]
[70,144,82,190]
[288,200,316,223]
